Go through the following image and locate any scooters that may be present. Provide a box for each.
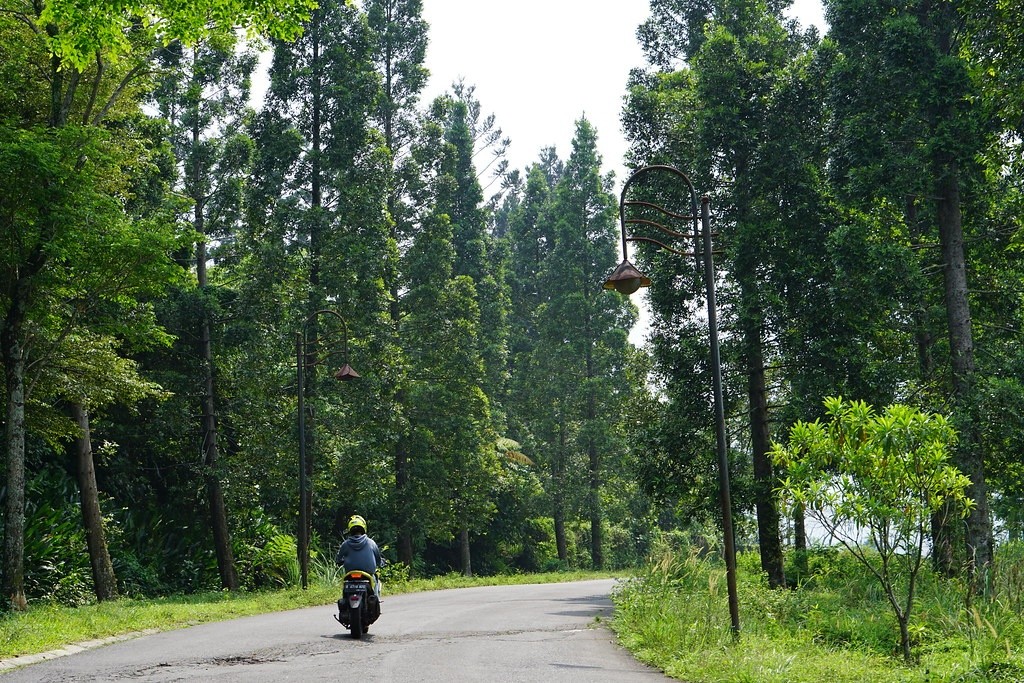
[333,569,381,639]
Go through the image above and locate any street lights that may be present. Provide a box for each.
[296,308,362,593]
[600,162,741,644]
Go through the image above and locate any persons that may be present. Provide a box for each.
[334,515,383,602]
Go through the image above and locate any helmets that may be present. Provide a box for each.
[347,515,366,533]
